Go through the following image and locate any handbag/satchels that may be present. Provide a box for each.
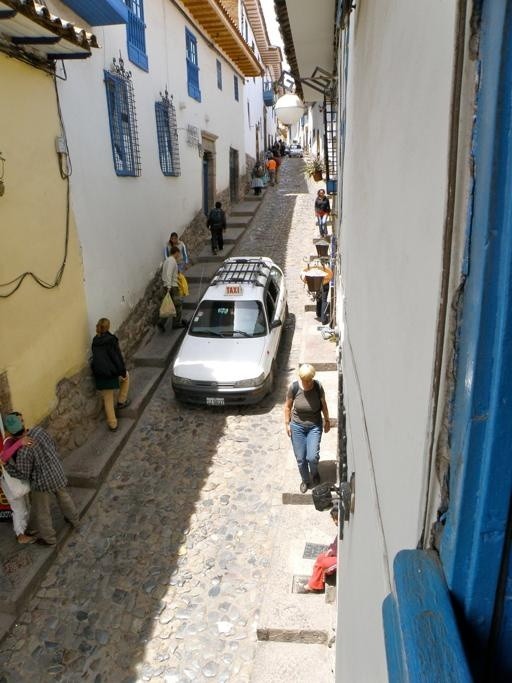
[4,472,31,498]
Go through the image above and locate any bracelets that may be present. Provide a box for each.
[325,420,330,422]
[283,362,331,493]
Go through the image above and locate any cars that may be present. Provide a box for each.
[172,256,286,407]
[284,145,303,158]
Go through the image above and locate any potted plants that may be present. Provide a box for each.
[301,159,326,181]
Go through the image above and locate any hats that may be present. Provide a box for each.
[5,413,23,433]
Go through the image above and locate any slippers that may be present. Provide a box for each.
[18,535,36,543]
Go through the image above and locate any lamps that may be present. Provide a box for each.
[315,233,339,262]
[312,471,356,520]
[274,74,339,128]
[299,254,342,300]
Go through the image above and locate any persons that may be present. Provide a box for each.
[303,506,338,592]
[153,246,187,331]
[264,139,286,157]
[206,200,227,255]
[0,412,41,545]
[90,317,132,431]
[267,157,277,186]
[260,163,270,186]
[301,256,333,325]
[0,414,81,545]
[314,189,331,238]
[250,161,265,195]
[163,231,189,273]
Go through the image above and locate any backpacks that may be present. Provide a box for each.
[211,208,222,230]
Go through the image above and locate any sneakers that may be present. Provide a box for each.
[117,398,131,408]
[298,583,323,594]
[300,480,308,492]
[214,248,217,255]
[63,516,80,527]
[312,472,320,484]
[173,322,185,329]
[109,414,120,432]
[35,537,52,545]
[157,323,165,331]
[219,246,224,250]
